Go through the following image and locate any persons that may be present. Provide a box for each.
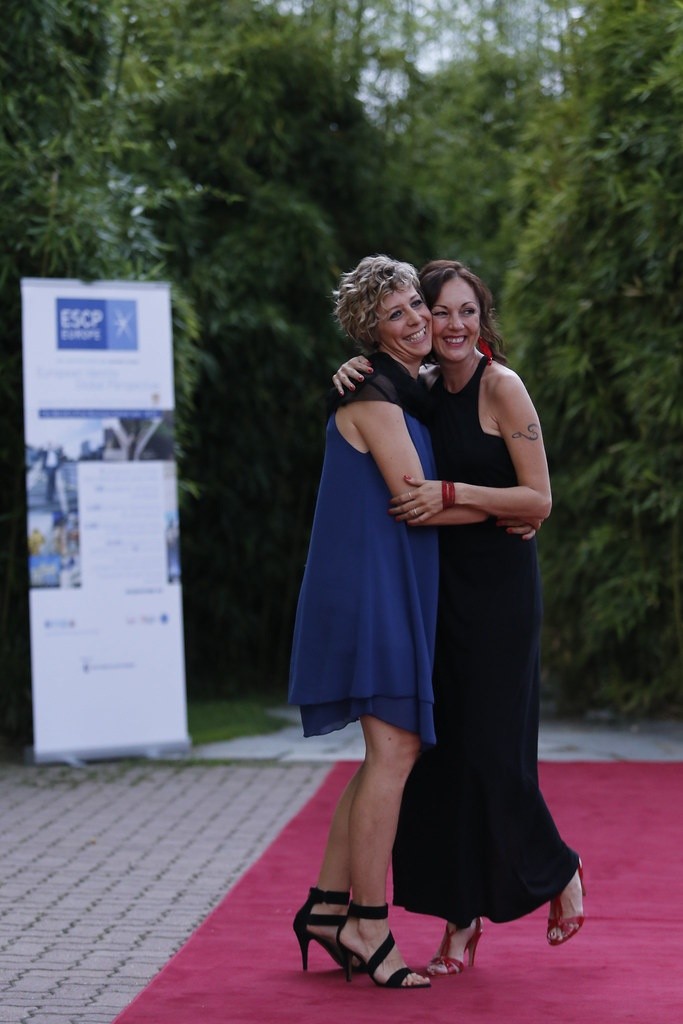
[288,256,543,988]
[332,259,586,978]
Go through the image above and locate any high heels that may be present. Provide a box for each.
[547,855,586,946]
[427,915,484,976]
[336,900,432,988]
[293,885,368,973]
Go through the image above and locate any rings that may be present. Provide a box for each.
[408,492,412,500]
[414,508,418,516]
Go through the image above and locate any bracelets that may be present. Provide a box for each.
[440,481,455,510]
